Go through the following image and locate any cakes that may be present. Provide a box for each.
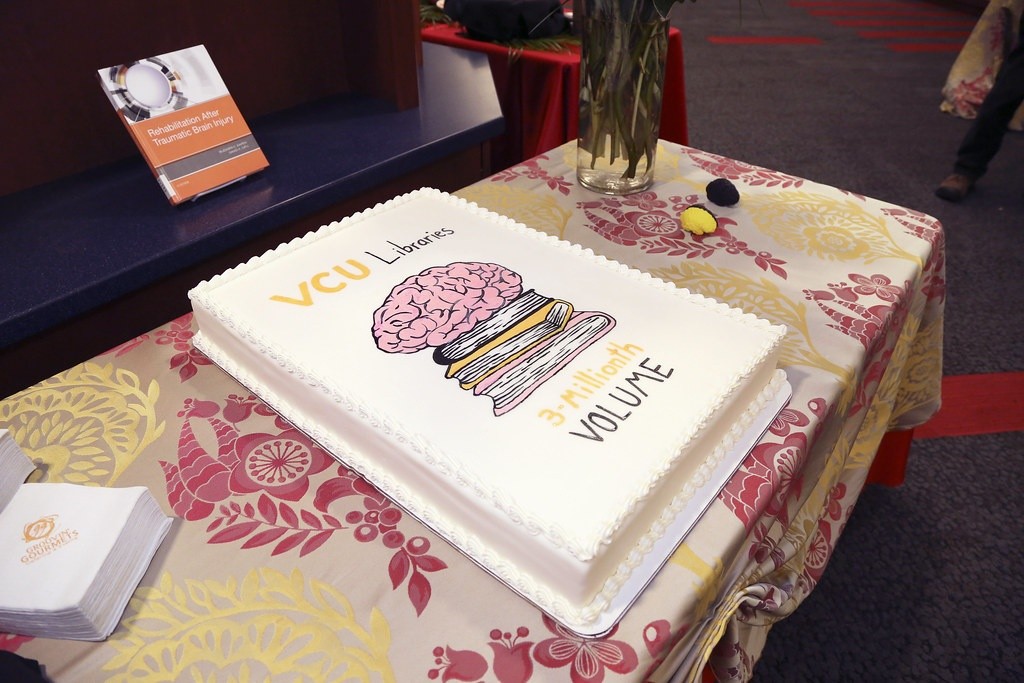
[186,188,788,626]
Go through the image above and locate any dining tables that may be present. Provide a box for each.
[0,125,941,683]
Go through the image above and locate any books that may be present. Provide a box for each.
[97,43,270,203]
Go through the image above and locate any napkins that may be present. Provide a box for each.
[0,425,175,643]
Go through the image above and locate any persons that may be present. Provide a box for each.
[934,10,1024,203]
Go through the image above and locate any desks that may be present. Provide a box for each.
[421,0,690,159]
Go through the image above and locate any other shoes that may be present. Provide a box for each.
[934,172,971,203]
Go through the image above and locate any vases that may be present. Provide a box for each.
[576,0,671,195]
